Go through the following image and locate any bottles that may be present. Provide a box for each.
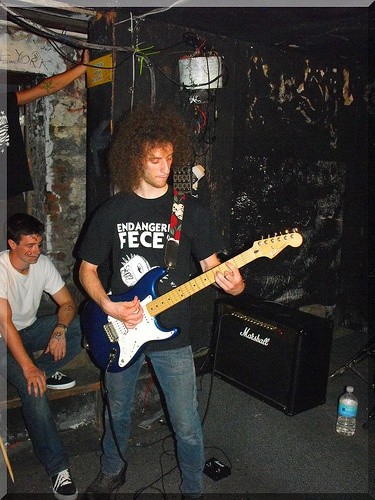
[336,386,358,436]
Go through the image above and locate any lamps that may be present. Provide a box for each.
[177,52,225,114]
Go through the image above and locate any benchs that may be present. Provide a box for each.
[0,370,158,457]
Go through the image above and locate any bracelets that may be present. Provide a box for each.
[57,324,68,333]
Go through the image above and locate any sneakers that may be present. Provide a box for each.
[49,466,78,500]
[44,369,76,390]
[83,463,128,500]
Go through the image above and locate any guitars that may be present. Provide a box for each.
[82,228,302,372]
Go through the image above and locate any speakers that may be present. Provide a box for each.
[210,295,334,416]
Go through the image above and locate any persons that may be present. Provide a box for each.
[0,214,77,500]
[72,111,245,499]
